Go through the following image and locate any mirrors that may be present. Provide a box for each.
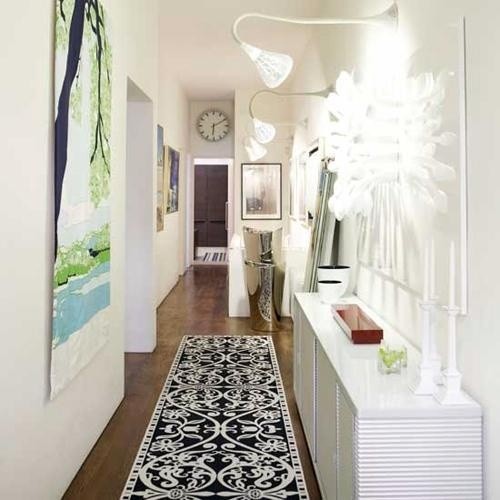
[351,21,466,313]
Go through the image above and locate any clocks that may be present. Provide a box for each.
[197,108,230,141]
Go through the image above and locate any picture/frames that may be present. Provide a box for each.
[240,163,282,221]
[157,124,181,235]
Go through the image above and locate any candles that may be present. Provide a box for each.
[419,238,459,305]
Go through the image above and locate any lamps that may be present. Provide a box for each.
[238,2,404,160]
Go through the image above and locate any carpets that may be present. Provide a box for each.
[118,330,309,499]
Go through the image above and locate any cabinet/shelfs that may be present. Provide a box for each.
[293,294,484,500]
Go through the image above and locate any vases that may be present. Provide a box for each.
[316,264,352,303]
